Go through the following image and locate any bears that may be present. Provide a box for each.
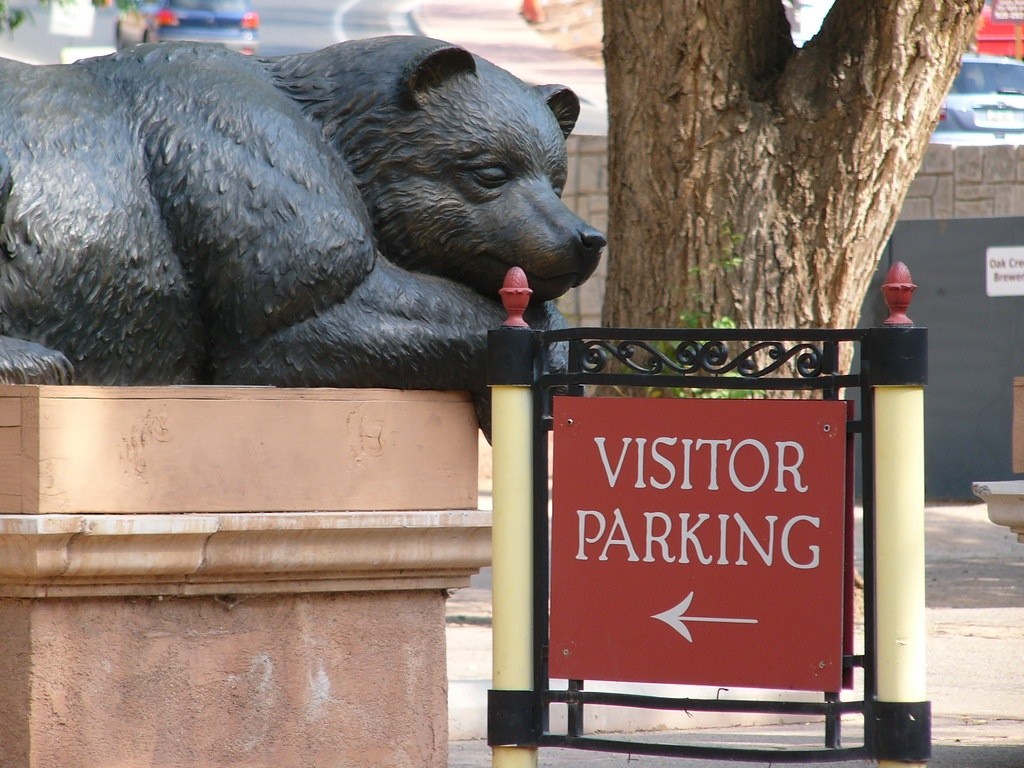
[0,33,606,449]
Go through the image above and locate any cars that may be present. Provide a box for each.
[925,53,1024,148]
[113,0,261,59]
